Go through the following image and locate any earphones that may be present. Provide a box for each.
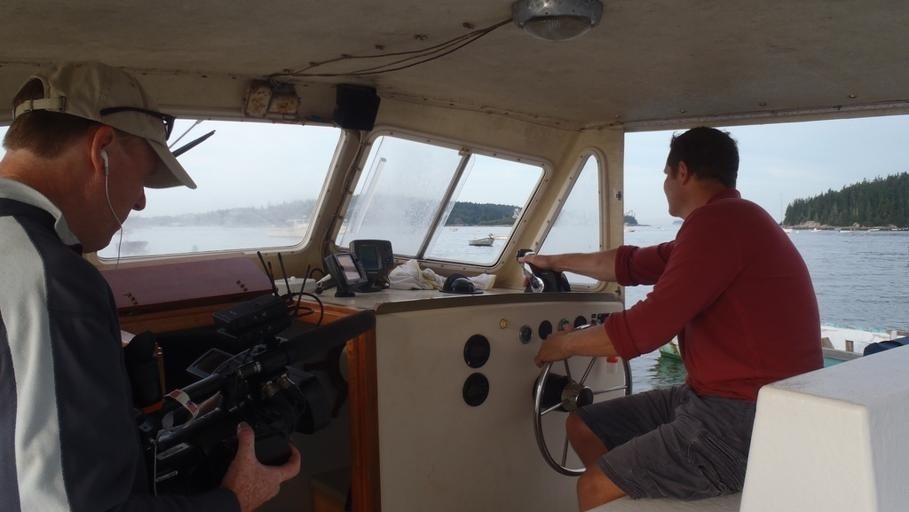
[99,148,111,176]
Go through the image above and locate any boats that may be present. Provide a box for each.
[117,230,148,251]
[469,233,494,246]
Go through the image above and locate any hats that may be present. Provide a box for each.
[11,60,197,189]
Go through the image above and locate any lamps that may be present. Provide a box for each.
[510,0,603,43]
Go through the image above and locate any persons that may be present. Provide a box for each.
[515,124,825,510]
[1,60,304,512]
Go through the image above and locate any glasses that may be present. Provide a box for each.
[100,106,176,141]
